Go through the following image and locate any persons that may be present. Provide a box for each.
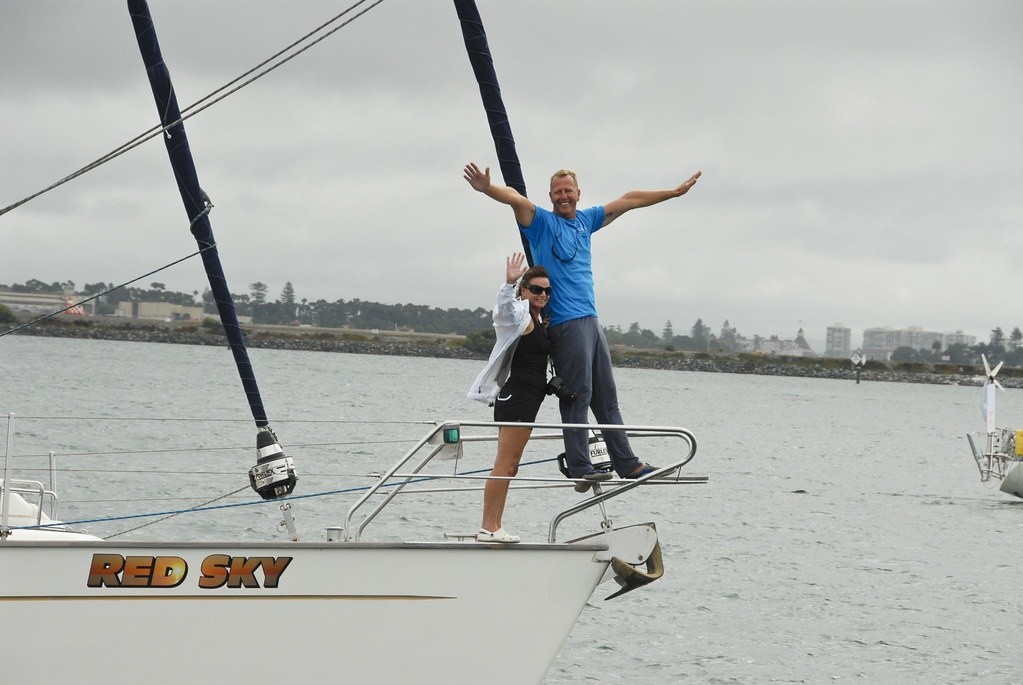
[467,253,552,542]
[462,162,702,493]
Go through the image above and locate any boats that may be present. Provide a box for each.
[966,353,1023,504]
[1,1,709,685]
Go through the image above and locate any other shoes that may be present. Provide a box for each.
[626,463,676,479]
[575,470,613,493]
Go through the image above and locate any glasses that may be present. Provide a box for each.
[523,284,552,295]
[552,233,577,262]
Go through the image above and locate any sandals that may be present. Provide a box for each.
[478,527,520,543]
